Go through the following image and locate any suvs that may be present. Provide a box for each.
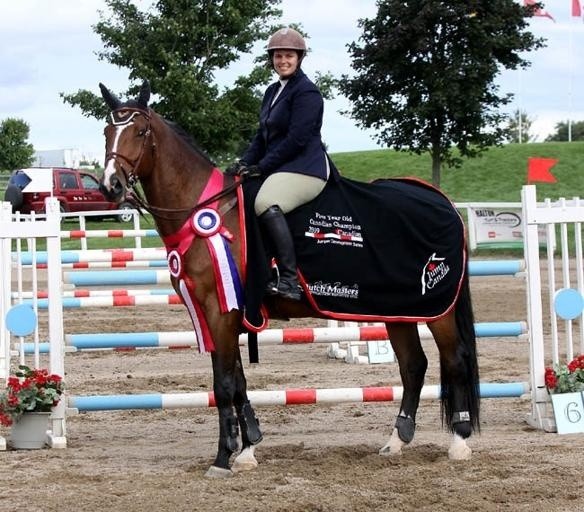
[3,165,141,227]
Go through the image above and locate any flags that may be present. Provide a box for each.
[524,0,555,22]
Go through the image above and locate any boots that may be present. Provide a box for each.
[257,204,301,301]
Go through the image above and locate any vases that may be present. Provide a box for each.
[9,413,52,449]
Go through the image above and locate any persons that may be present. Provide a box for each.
[224,27,332,300]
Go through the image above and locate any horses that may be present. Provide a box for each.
[98,80,483,481]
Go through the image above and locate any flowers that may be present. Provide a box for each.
[543,354,584,395]
[0,362,66,429]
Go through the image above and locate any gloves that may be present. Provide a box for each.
[225,160,262,177]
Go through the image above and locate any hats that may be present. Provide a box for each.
[266,28,307,51]
